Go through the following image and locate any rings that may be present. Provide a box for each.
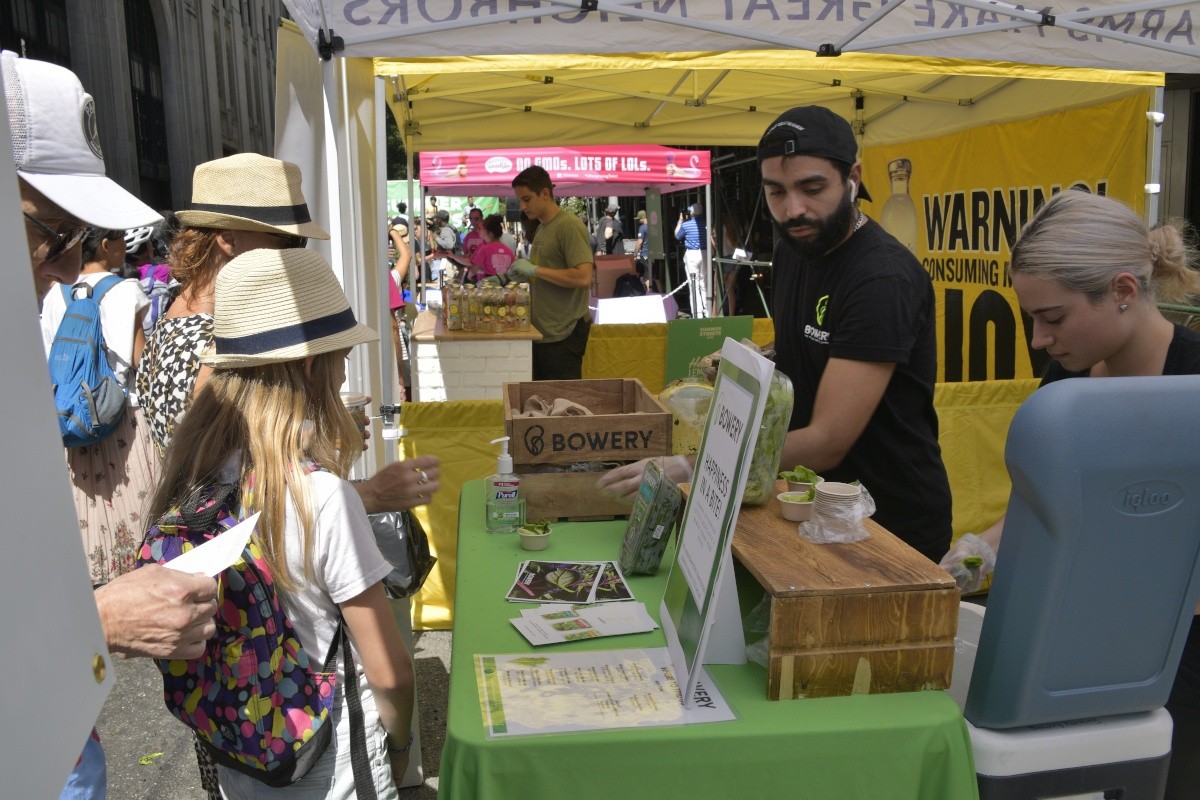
[420,470,428,484]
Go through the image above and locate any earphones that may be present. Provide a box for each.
[848,179,856,204]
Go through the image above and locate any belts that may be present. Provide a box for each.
[685,247,706,250]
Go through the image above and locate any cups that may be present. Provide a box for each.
[517,527,552,550]
[332,392,367,449]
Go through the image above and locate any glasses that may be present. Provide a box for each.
[150,237,159,247]
[469,217,478,221]
[21,209,92,263]
[266,232,308,248]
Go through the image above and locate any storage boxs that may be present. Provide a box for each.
[947,374,1200,800]
[617,460,682,577]
[502,378,672,523]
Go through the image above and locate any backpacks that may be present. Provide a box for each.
[390,214,411,244]
[46,272,125,447]
[138,263,173,344]
[730,220,761,261]
[614,272,646,297]
[439,223,462,257]
[133,447,378,800]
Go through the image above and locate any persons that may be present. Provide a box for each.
[504,167,593,379]
[0,45,439,799]
[593,106,953,572]
[940,189,1200,598]
[384,191,772,402]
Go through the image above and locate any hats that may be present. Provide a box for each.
[721,198,744,212]
[198,247,381,369]
[687,203,703,215]
[605,203,620,213]
[434,210,449,218]
[634,210,646,219]
[174,152,331,241]
[756,105,872,202]
[580,216,588,223]
[431,197,436,200]
[0,50,165,232]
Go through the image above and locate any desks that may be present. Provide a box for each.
[410,310,544,401]
[438,479,981,800]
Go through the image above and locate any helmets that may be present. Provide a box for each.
[124,226,154,254]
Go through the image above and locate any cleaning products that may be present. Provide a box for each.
[483,433,528,531]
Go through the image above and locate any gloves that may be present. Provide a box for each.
[507,259,538,283]
[482,272,506,286]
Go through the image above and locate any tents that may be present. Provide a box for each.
[419,145,712,319]
[368,46,1167,556]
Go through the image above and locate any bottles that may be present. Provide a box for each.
[442,278,530,332]
[880,159,917,259]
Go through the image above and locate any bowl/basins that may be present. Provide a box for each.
[813,482,861,521]
[776,491,813,521]
[786,476,825,492]
